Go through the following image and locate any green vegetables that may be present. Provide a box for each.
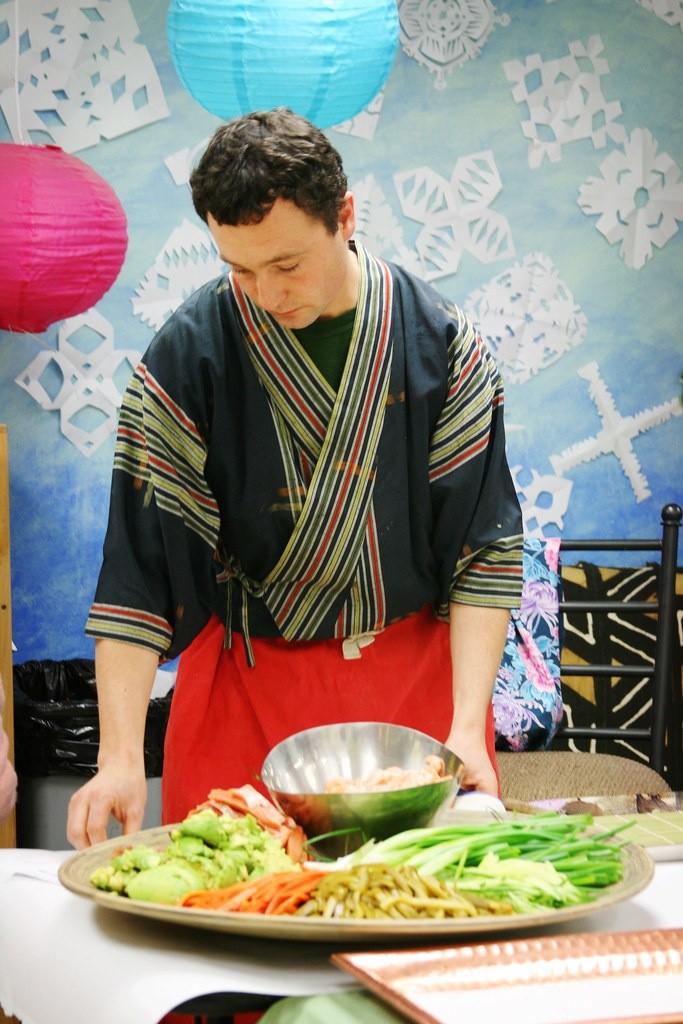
[90,813,293,910]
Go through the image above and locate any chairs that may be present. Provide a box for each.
[492,504,683,801]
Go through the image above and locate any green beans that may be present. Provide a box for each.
[314,863,512,923]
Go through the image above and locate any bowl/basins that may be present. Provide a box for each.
[260,720,466,863]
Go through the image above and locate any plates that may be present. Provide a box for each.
[57,811,656,944]
[331,928,683,1024]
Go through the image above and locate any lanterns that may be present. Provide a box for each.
[0,143,127,333]
[167,0,401,129]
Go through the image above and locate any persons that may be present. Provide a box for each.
[67,107,523,851]
[0,715,17,818]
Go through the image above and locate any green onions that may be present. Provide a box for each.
[338,811,636,885]
